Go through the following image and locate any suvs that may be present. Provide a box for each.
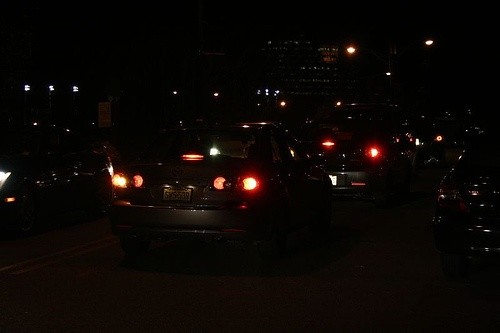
[318,99,413,205]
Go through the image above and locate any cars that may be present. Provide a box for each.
[100,121,334,245]
[0,140,91,236]
[434,138,500,276]
